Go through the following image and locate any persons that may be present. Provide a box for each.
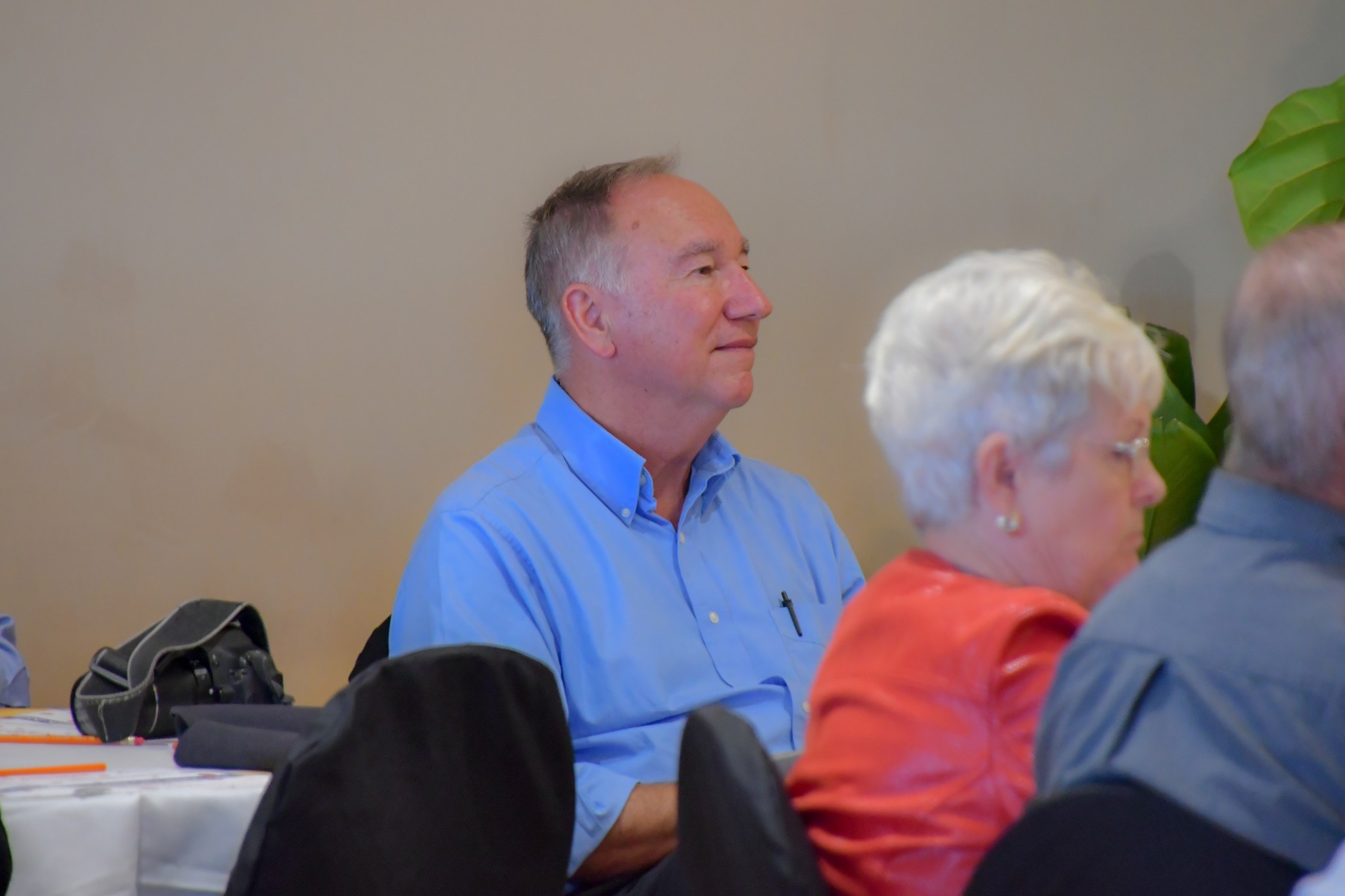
[1032,223,1345,896]
[784,247,1167,896]
[387,158,866,879]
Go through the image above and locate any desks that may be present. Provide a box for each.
[1,707,278,895]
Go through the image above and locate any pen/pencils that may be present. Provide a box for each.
[0,735,143,745]
[781,590,802,636]
[0,764,107,775]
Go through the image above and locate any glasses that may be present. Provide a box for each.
[1036,430,1151,471]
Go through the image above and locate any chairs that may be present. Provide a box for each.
[679,707,836,894]
[224,643,577,896]
